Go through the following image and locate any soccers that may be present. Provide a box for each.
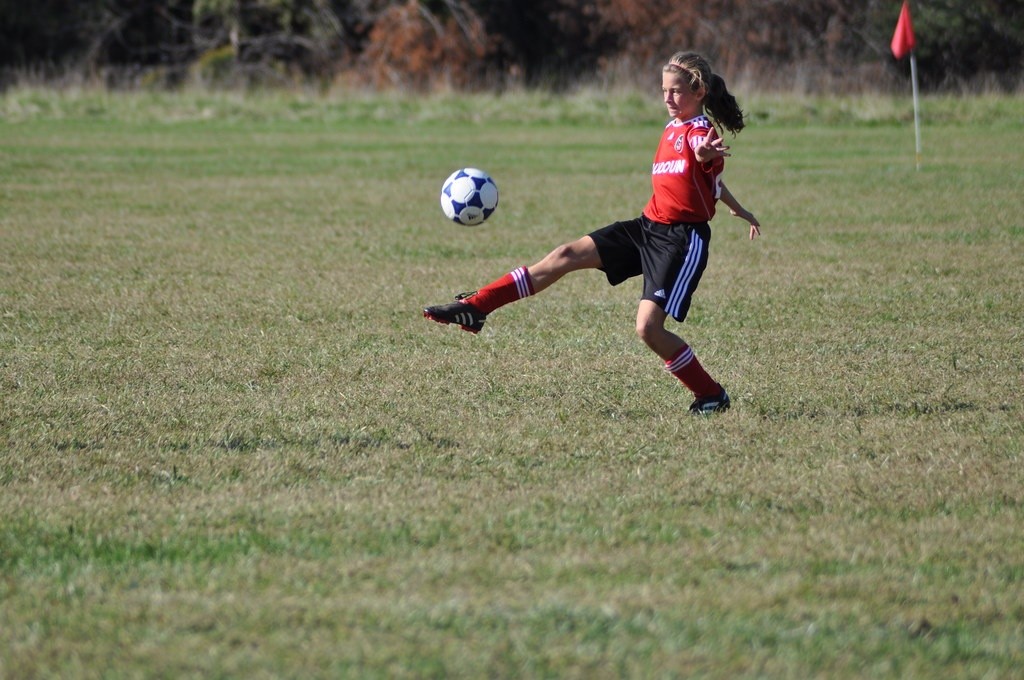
[440,166,501,224]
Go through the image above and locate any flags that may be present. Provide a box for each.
[889,0,914,58]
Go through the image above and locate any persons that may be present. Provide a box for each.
[423,49,763,417]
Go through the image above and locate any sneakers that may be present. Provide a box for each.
[422,297,486,334]
[686,385,731,417]
